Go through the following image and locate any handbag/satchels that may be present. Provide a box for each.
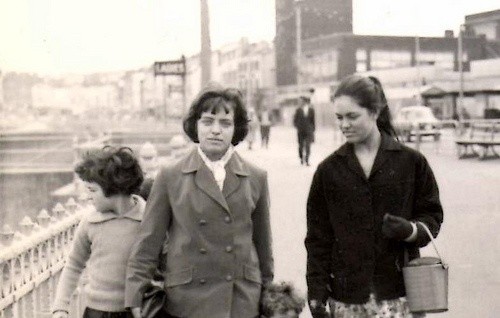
[402,222,450,316]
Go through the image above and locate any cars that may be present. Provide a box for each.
[391,106,443,143]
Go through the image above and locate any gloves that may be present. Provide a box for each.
[309,299,329,318]
[382,213,413,242]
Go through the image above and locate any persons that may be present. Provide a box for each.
[51,146,167,318]
[257,105,273,148]
[245,106,257,149]
[293,95,316,167]
[303,74,445,318]
[125,87,275,318]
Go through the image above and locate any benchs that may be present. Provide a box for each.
[452,120,500,159]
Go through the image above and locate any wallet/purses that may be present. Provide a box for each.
[140,285,168,318]
